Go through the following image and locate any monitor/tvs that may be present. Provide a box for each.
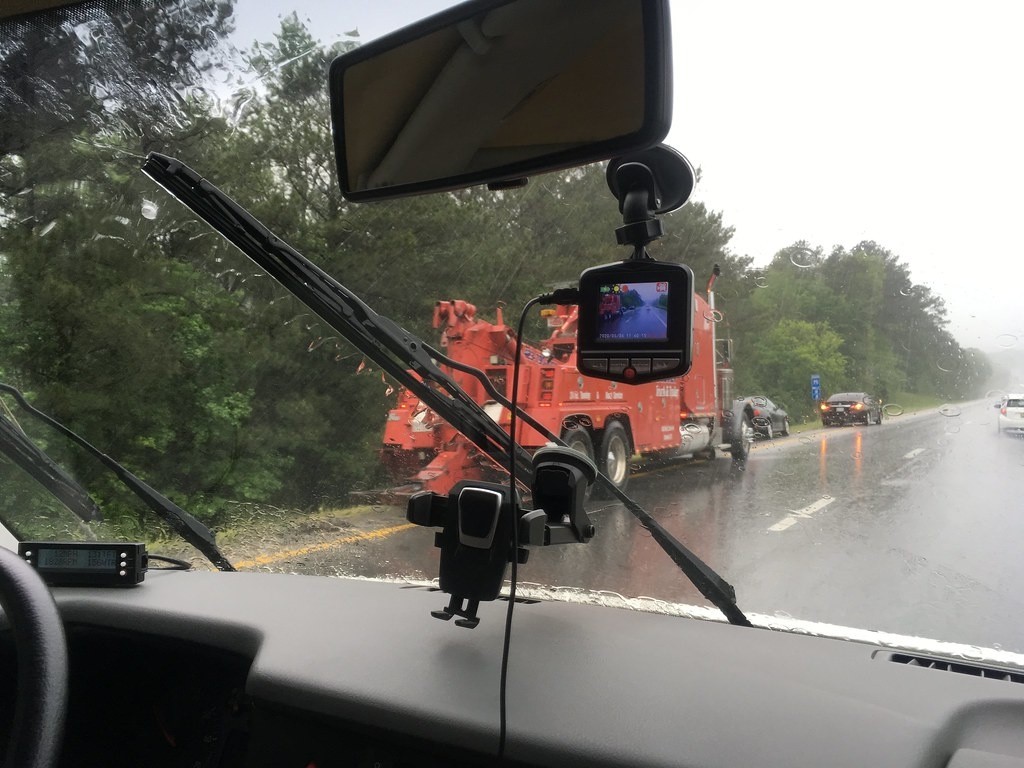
[576,261,694,382]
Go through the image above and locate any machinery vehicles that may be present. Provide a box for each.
[346,256,750,506]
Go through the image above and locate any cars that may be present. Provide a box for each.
[821,392,883,427]
[745,396,789,442]
[994,394,1024,434]
